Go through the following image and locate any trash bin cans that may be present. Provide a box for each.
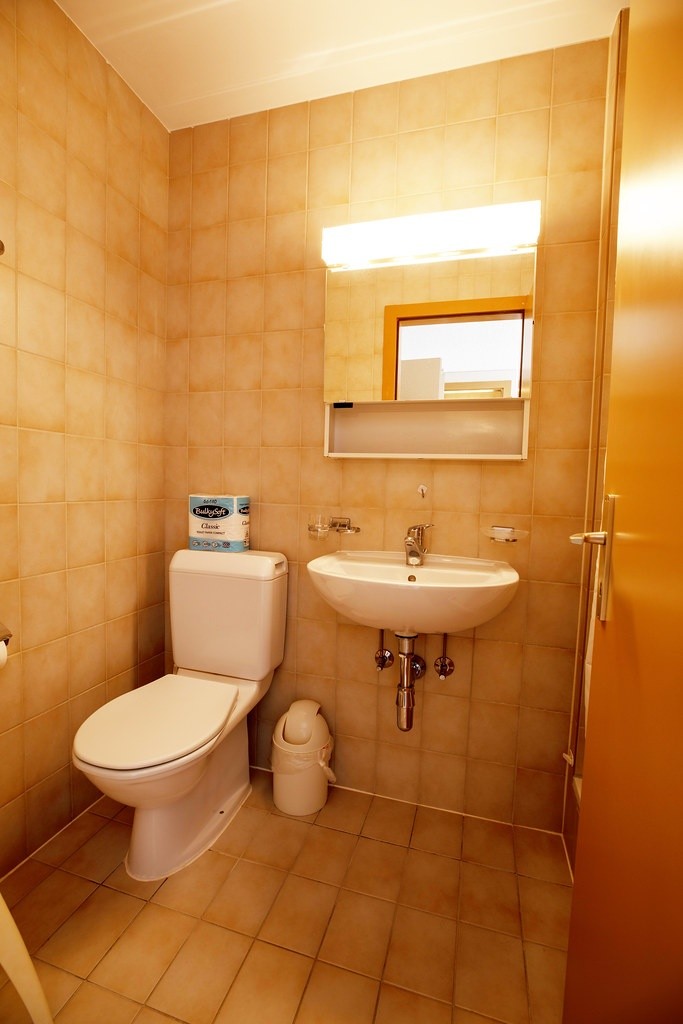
[271,699,337,817]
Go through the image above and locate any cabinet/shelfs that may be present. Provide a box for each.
[322,395,531,464]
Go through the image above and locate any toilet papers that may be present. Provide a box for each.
[187,491,252,550]
[0,640,8,670]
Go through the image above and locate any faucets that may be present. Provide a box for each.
[403,522,435,566]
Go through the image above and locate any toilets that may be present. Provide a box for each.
[69,549,290,883]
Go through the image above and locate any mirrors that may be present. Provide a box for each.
[321,247,538,404]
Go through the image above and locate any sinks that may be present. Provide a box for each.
[304,547,520,636]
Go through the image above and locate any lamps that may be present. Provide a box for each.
[320,200,542,267]
